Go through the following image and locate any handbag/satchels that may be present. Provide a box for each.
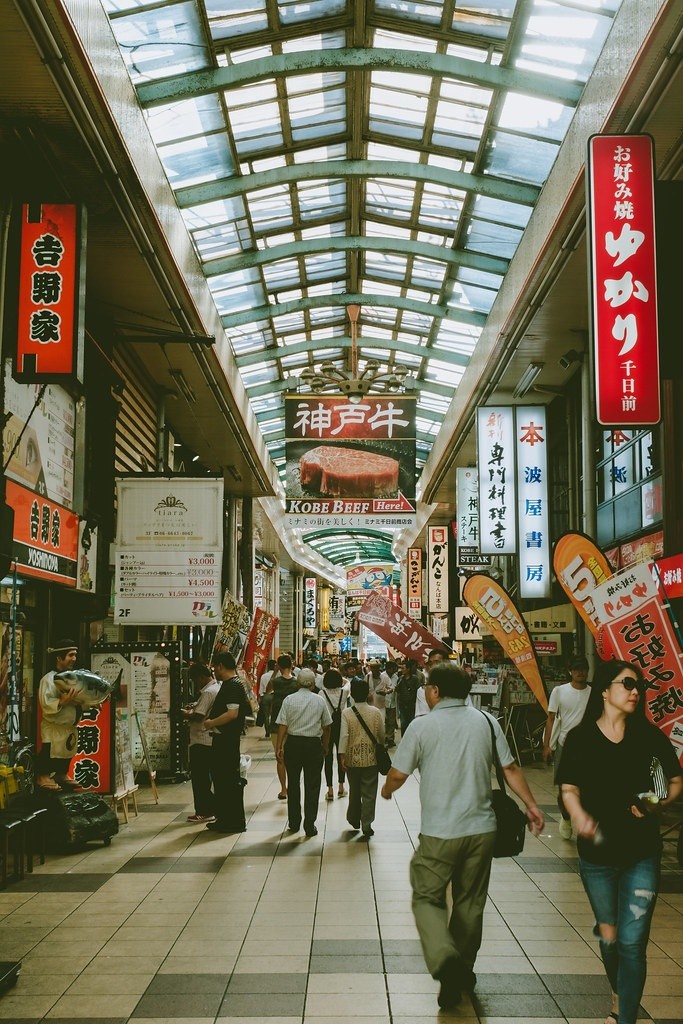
[542,686,561,751]
[491,789,529,858]
[256,709,264,727]
[240,754,251,778]
[649,757,668,799]
[375,744,392,775]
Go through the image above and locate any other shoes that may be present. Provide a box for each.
[559,816,573,839]
[207,822,231,833]
[438,956,464,1009]
[363,829,374,836]
[265,727,270,738]
[306,826,318,836]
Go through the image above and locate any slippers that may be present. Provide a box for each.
[338,788,348,796]
[278,793,286,799]
[325,792,334,800]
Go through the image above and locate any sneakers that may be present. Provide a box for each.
[188,815,215,822]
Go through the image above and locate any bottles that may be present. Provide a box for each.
[642,794,660,813]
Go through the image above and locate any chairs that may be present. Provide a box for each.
[0,766,49,890]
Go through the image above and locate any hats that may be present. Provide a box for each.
[570,656,589,670]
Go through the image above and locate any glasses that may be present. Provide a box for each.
[610,676,643,696]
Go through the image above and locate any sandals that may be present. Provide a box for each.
[604,1011,619,1024]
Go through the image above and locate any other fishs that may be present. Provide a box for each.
[53,667,124,705]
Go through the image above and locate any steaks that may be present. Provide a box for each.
[300,446,400,499]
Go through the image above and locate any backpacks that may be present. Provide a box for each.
[323,689,344,741]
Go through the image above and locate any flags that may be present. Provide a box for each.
[213,531,683,767]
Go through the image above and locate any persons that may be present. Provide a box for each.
[32,639,102,791]
[179,659,220,821]
[546,656,592,839]
[382,663,544,1012]
[554,660,683,1024]
[205,652,249,833]
[276,668,334,836]
[259,648,472,801]
[338,679,384,835]
